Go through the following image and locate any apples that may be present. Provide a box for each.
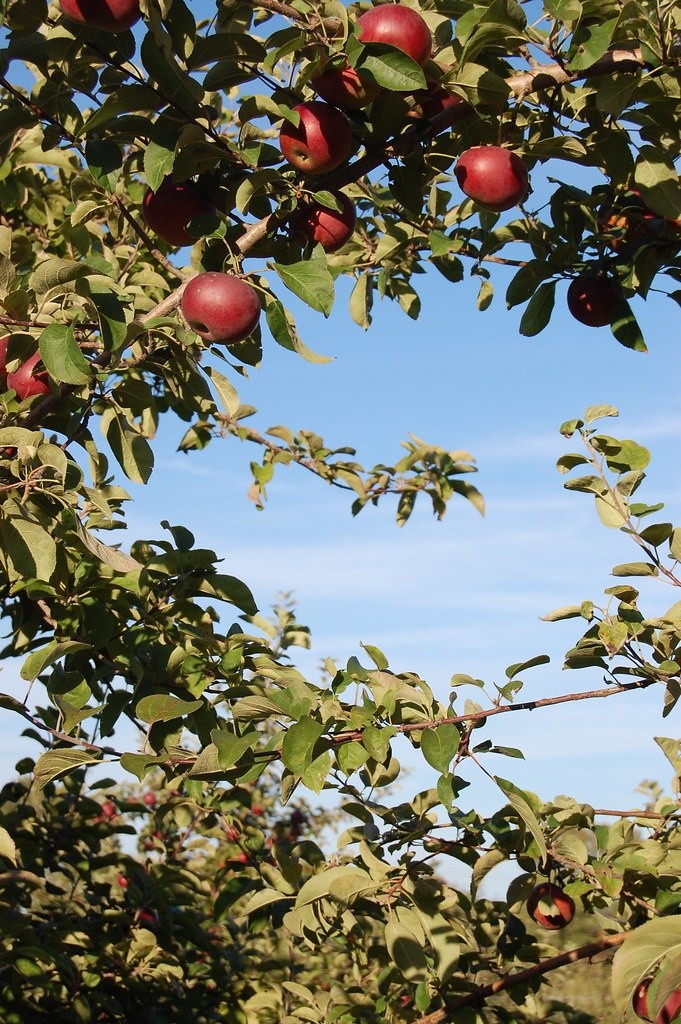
[181,271,261,344]
[0,334,57,402]
[142,176,217,246]
[527,882,575,930]
[58,0,143,32]
[633,978,681,1024]
[91,790,301,932]
[278,4,464,175]
[455,145,528,212]
[567,185,681,326]
[289,191,356,253]
